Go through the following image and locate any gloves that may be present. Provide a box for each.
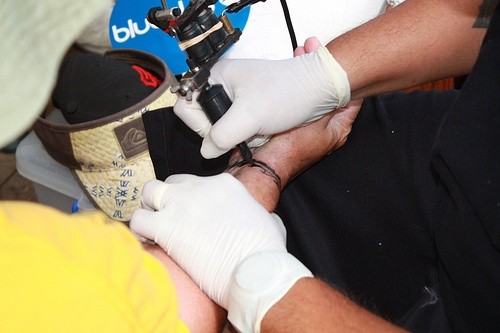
[129,172,316,333]
[173,45,354,159]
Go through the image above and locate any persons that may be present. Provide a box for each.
[128,0,499,333]
[0,35,362,333]
